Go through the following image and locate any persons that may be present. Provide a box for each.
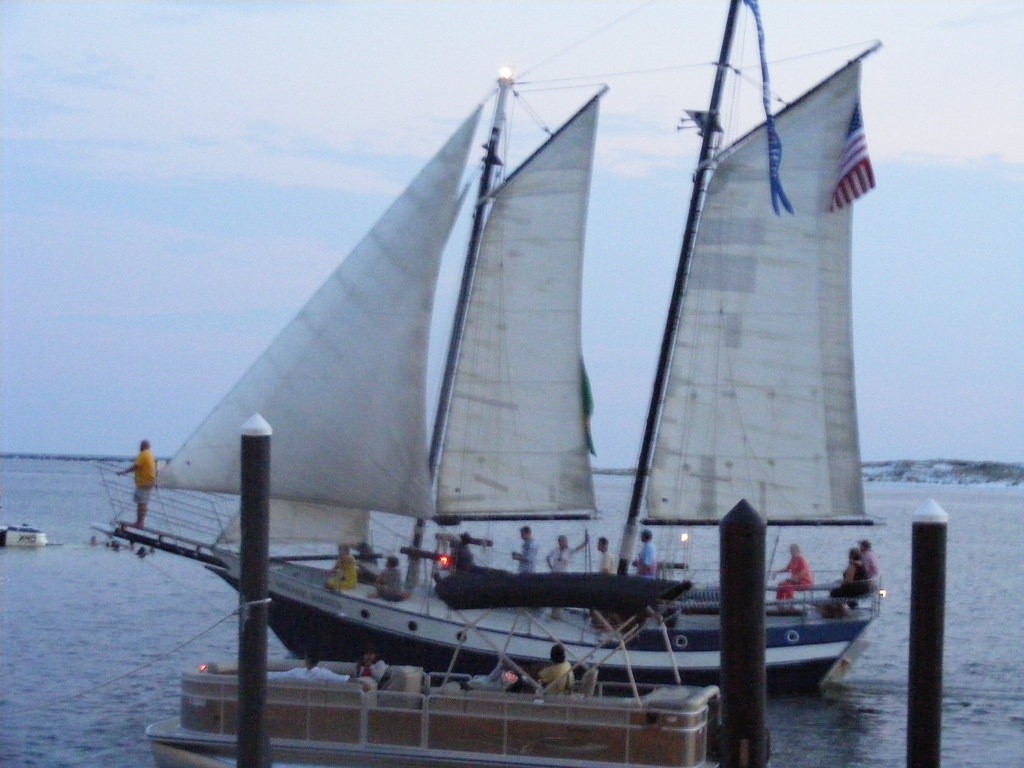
[857,539,876,577]
[369,555,401,600]
[450,540,473,564]
[597,537,614,573]
[267,650,351,681]
[775,544,811,599]
[830,548,870,608]
[356,641,386,682]
[118,440,156,528]
[511,527,538,574]
[539,644,574,696]
[631,529,657,576]
[328,545,358,591]
[352,542,378,584]
[547,535,585,572]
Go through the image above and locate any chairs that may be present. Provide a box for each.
[317,661,361,679]
[346,682,364,692]
[356,676,377,691]
[378,665,403,691]
[208,660,239,675]
[268,659,307,672]
[580,668,599,698]
[386,666,423,692]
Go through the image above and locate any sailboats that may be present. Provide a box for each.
[89,0,887,696]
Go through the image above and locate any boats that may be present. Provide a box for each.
[145,570,722,768]
